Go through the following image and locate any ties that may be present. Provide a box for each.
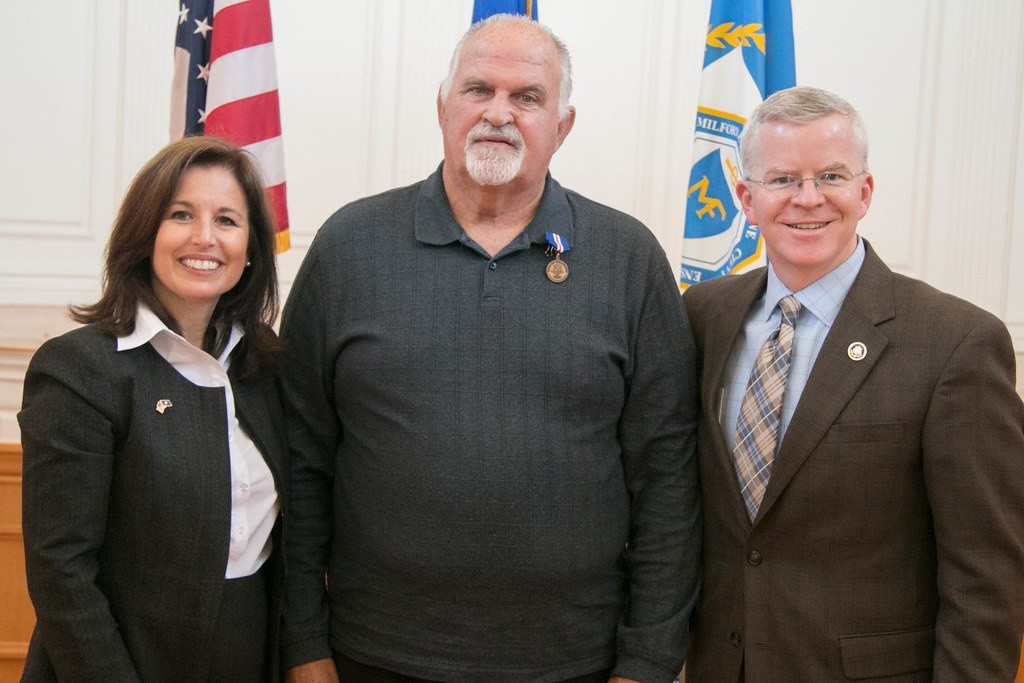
[730,296,802,524]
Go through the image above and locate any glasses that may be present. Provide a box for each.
[745,170,866,195]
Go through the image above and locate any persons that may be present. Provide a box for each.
[683,86,1023,683]
[15,133,291,683]
[279,11,703,682]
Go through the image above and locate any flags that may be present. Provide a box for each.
[470,0,540,24]
[168,0,292,253]
[679,0,798,294]
